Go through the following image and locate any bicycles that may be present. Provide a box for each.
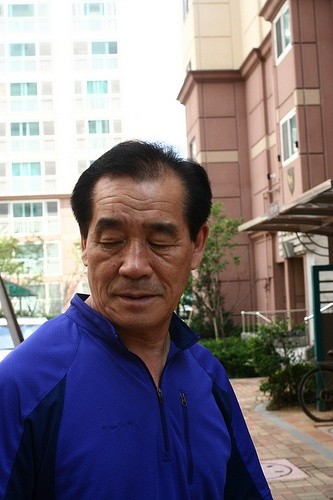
[296,349,332,423]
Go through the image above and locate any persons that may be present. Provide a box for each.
[0,139,274,500]
